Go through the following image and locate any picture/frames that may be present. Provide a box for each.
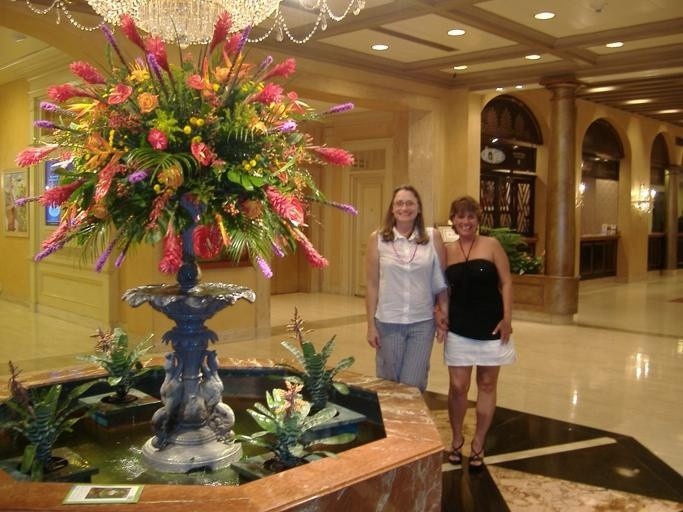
[0,169,30,238]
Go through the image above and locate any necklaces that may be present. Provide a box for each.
[392,241,419,264]
[458,236,475,261]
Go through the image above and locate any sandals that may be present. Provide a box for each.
[449,436,485,471]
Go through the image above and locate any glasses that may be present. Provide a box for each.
[391,201,419,208]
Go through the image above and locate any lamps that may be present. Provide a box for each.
[26,0,366,48]
[576,181,586,208]
[631,184,656,213]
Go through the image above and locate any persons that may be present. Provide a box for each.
[434,194,513,473]
[365,185,449,393]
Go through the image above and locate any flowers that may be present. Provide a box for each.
[283,306,356,413]
[1,361,112,483]
[235,384,335,471]
[15,12,358,287]
[67,325,157,403]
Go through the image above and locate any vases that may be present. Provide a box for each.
[78,389,161,429]
[308,400,367,440]
[174,213,202,288]
[230,449,318,482]
[0,446,99,483]
